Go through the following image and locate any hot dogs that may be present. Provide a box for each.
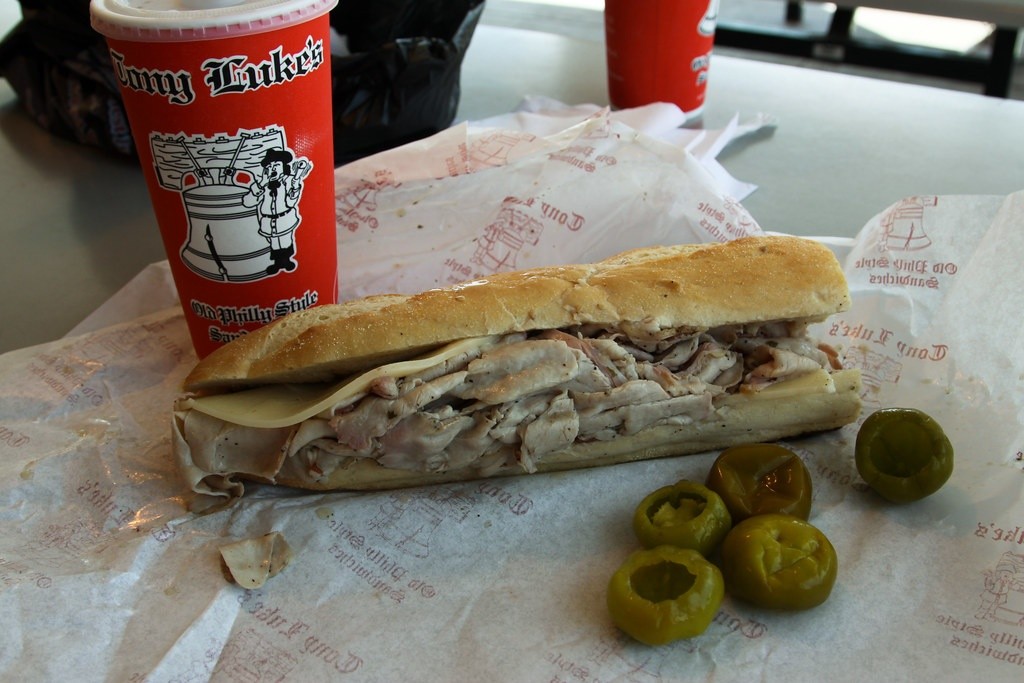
[168,234,861,516]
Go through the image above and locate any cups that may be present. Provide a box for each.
[604,1,718,124]
[89,0,339,362]
